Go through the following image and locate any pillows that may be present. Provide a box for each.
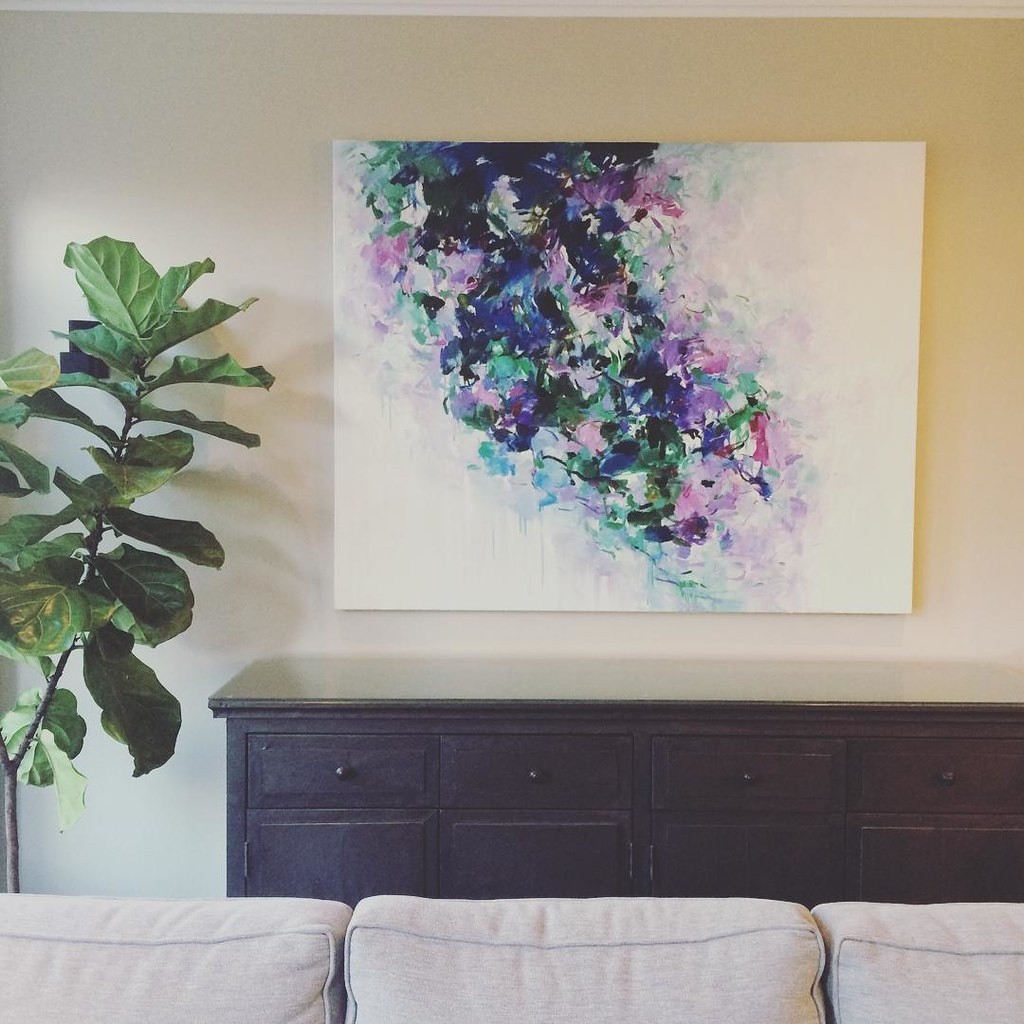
[342,894,829,1024]
[0,893,354,1024]
[810,900,1024,1024]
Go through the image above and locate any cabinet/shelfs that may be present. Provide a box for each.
[209,649,1024,911]
